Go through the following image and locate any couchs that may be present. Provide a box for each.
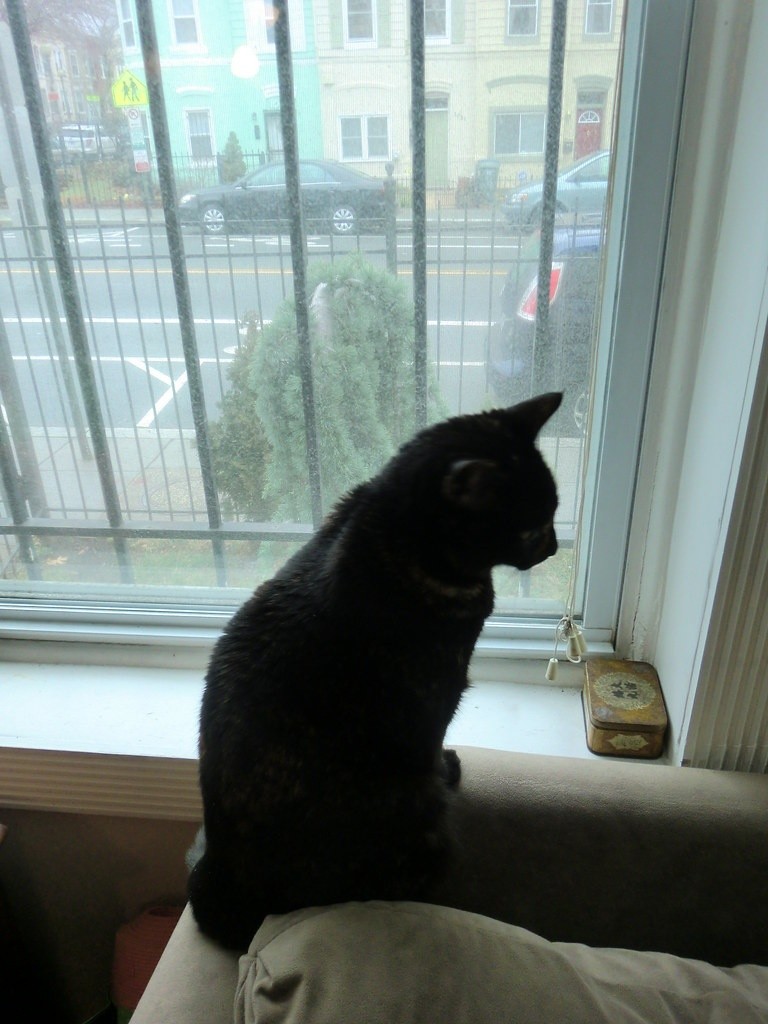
[128,745,768,1024]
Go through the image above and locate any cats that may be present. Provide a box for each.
[185,391,562,944]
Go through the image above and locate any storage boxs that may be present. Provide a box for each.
[581,657,670,759]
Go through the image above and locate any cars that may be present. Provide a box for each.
[179,160,392,236]
[503,149,615,227]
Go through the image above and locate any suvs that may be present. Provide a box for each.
[58,123,121,155]
[488,220,607,436]
